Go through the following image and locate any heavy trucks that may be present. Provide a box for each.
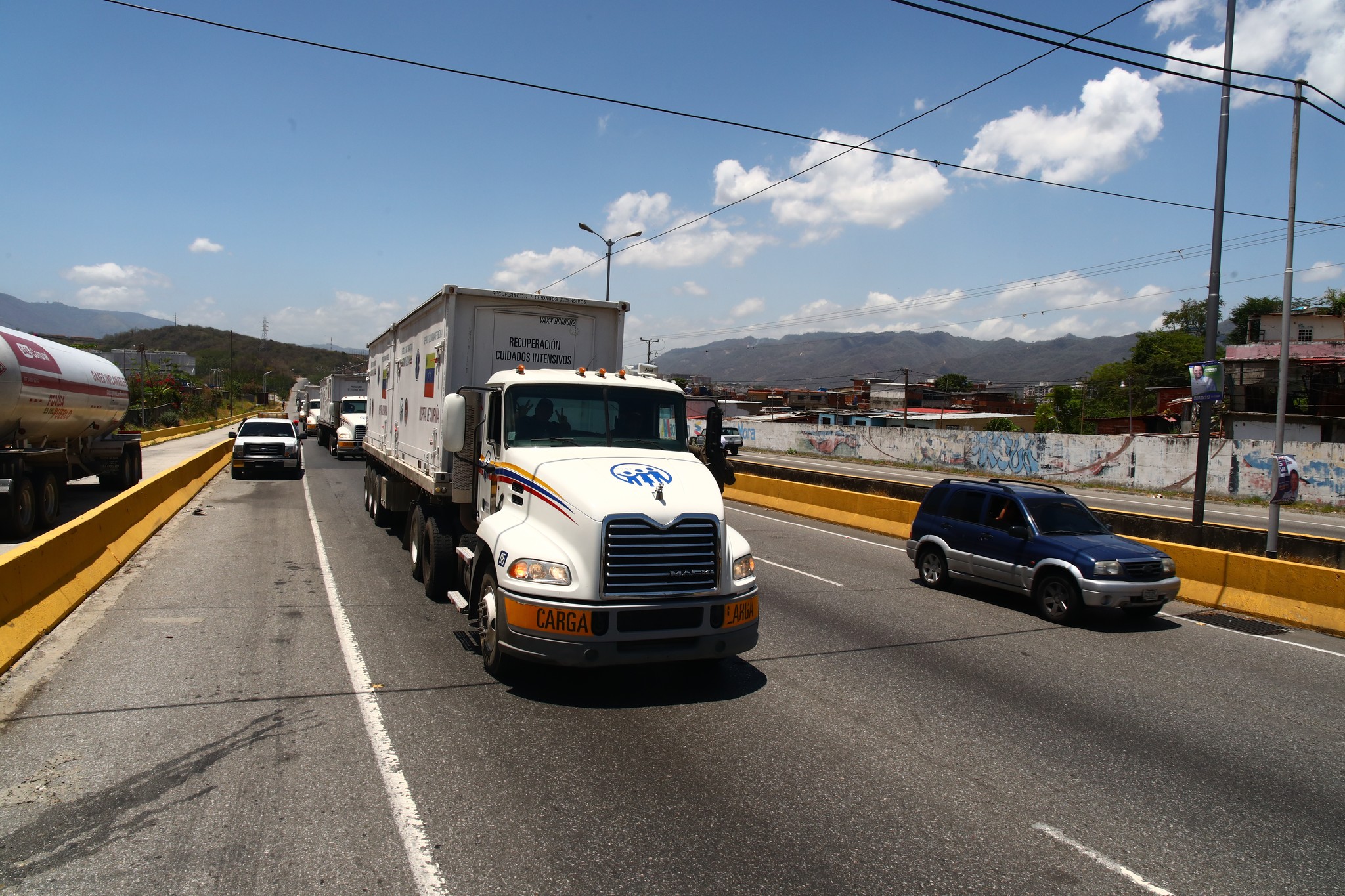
[295,384,320,436]
[317,374,369,460]
[0,325,144,540]
[363,283,760,678]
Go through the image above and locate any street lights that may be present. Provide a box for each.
[578,221,643,301]
[207,367,224,387]
[1119,375,1133,437]
[192,368,195,382]
[262,370,272,409]
[324,357,368,376]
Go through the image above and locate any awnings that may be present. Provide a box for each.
[1218,355,1345,367]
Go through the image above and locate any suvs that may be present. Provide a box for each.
[228,413,307,480]
[904,476,1182,623]
[689,427,743,455]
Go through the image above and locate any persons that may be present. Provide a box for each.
[282,402,285,409]
[1190,365,1216,395]
[702,434,706,436]
[688,435,736,496]
[998,498,1013,520]
[1281,469,1298,502]
[515,398,573,439]
